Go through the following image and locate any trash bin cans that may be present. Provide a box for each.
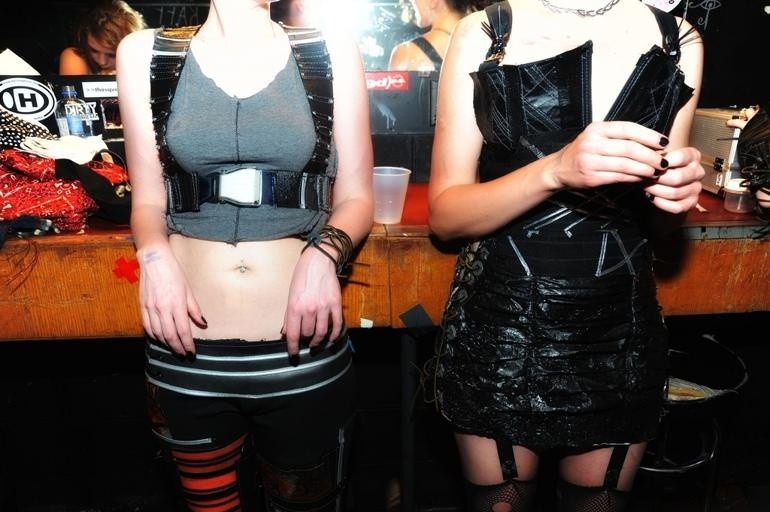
[637,332,748,512]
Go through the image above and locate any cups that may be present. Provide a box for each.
[371,166,413,224]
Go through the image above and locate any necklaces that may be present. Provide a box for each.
[433,25,450,36]
[539,0,623,17]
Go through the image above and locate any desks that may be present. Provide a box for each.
[0,182,770,341]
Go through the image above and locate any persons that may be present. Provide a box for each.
[59,1,148,77]
[115,0,376,511]
[428,0,706,509]
[387,0,497,72]
[726,102,770,209]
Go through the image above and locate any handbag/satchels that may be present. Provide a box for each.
[1,147,130,240]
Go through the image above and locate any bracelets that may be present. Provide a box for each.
[310,225,354,275]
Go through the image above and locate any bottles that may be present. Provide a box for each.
[53,84,93,137]
[722,178,759,215]
